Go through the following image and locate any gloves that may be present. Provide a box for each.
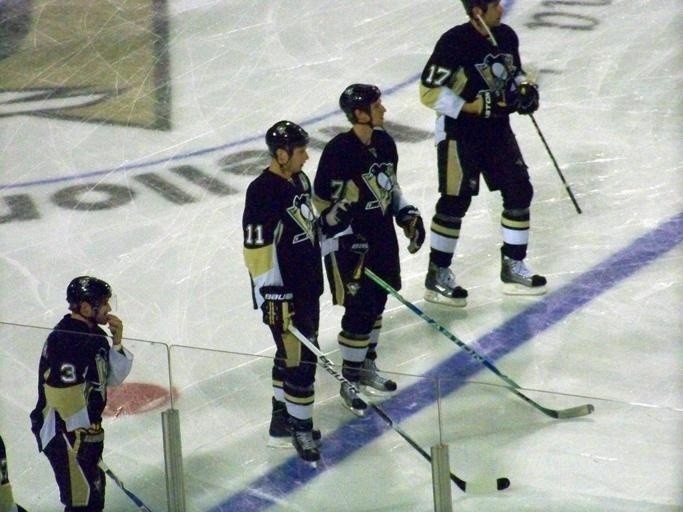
[397,205,425,253]
[259,286,296,334]
[346,235,368,289]
[77,431,104,478]
[320,202,352,236]
[509,84,538,116]
[477,88,509,119]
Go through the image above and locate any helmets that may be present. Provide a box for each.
[339,85,381,123]
[66,277,111,311]
[266,120,310,153]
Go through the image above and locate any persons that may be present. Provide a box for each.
[0,436,21,512]
[314,84,425,414]
[30,277,134,512]
[242,121,358,468]
[420,0,548,308]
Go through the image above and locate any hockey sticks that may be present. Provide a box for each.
[476,13,582,215]
[96,457,151,512]
[363,266,595,420]
[287,323,512,497]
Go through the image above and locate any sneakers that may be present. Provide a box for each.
[424,255,468,298]
[359,362,395,391]
[267,400,322,439]
[498,245,547,287]
[292,418,322,461]
[339,382,368,411]
